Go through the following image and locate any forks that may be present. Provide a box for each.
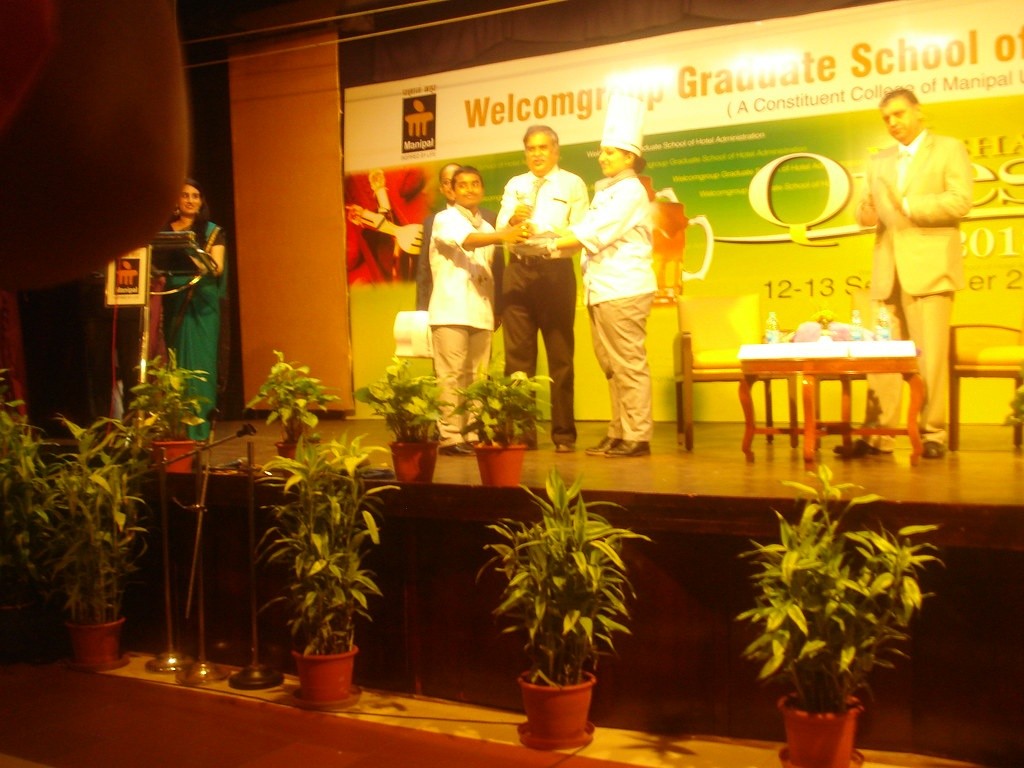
[346,203,424,256]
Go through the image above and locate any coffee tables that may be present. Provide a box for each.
[741,340,929,474]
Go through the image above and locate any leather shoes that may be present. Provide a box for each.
[438,444,475,456]
[585,434,650,457]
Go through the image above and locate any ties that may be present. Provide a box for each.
[529,178,546,208]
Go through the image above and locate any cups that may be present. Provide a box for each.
[594,174,681,202]
[649,202,713,309]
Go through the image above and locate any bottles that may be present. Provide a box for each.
[876,308,889,340]
[764,311,780,343]
[851,308,863,341]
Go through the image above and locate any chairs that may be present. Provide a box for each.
[670,320,866,451]
[948,322,1024,451]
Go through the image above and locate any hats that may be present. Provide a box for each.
[599,93,646,157]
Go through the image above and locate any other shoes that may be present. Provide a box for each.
[922,441,944,458]
[833,438,893,455]
[555,439,576,452]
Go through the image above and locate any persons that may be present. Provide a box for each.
[158,180,227,447]
[833,87,973,459]
[416,117,660,458]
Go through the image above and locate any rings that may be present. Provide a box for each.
[522,231,527,236]
[520,225,526,229]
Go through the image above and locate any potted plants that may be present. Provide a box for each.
[245,351,343,484]
[130,345,207,473]
[0,414,151,671]
[735,464,945,768]
[447,348,556,489]
[355,357,457,484]
[475,472,656,751]
